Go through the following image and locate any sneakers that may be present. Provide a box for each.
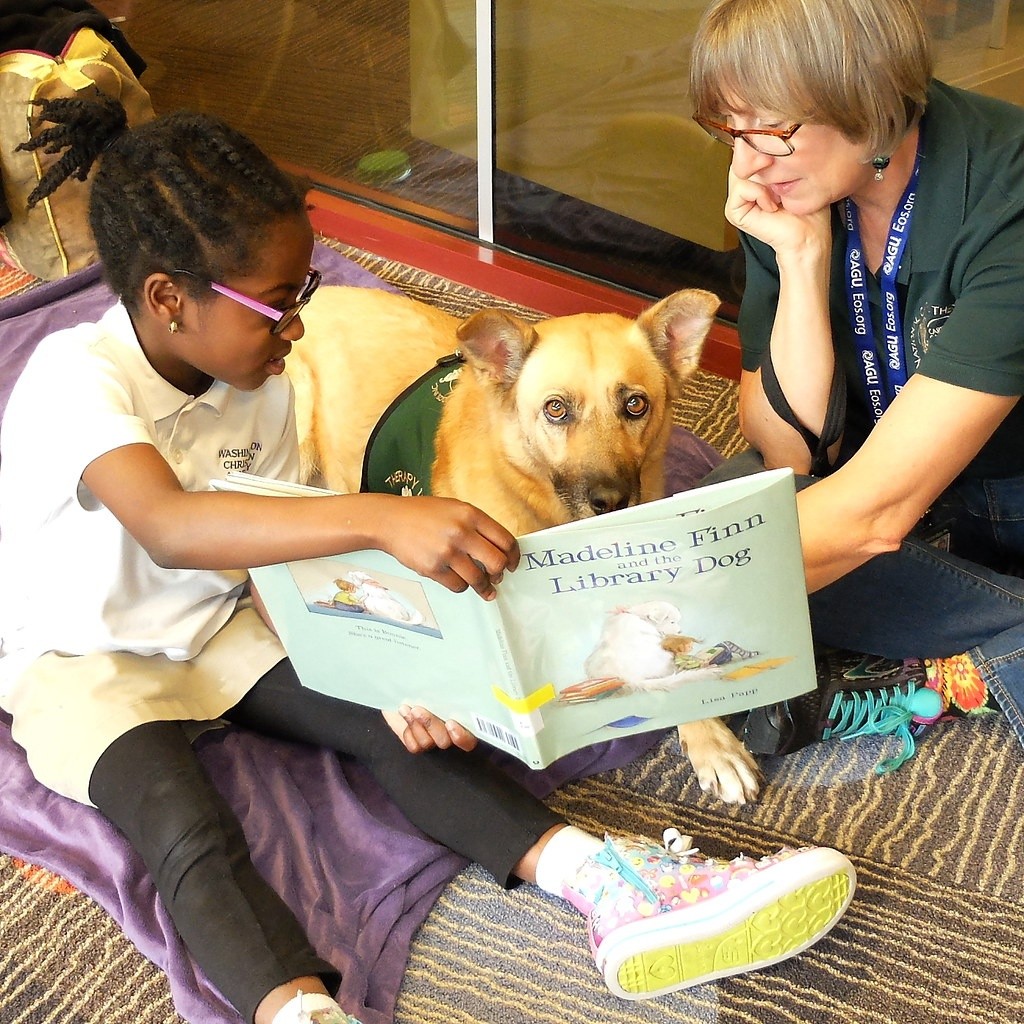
[563,827,857,1000]
[745,652,943,774]
[294,988,363,1024]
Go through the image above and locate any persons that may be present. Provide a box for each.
[686,0,1023,756]
[0,83,854,1024]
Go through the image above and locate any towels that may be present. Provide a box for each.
[1,230,727,1024]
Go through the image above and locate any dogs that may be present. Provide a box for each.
[279,285,770,805]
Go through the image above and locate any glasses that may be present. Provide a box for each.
[168,265,323,336]
[692,106,815,157]
